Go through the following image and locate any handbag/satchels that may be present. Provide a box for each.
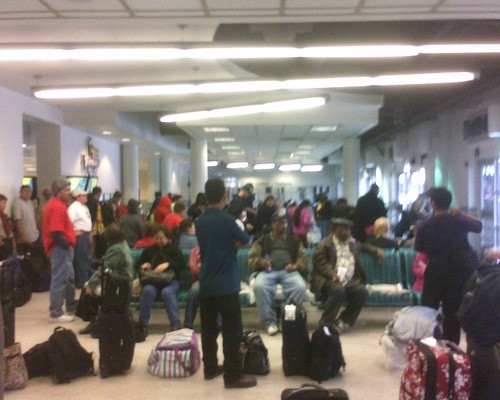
[140,255,175,285]
[456,265,500,346]
[400,339,472,400]
[276,299,310,376]
[75,287,98,321]
[228,331,269,375]
[3,326,28,390]
[310,326,346,383]
[148,328,200,377]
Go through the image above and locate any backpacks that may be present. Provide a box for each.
[50,327,97,384]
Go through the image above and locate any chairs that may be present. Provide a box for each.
[402,246,423,307]
[129,248,163,301]
[359,249,414,306]
[236,250,251,305]
[304,248,316,282]
[172,248,193,301]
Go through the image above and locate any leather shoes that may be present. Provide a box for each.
[225,378,256,388]
[79,322,91,334]
[205,365,224,380]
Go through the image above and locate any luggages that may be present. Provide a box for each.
[100,257,135,378]
[467,343,500,400]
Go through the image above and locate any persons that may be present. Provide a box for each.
[286,199,313,249]
[88,185,104,237]
[412,252,428,307]
[76,222,134,335]
[354,182,427,262]
[38,187,52,232]
[118,199,148,244]
[414,187,482,347]
[102,189,122,226]
[42,180,80,317]
[0,195,17,259]
[144,192,205,234]
[183,246,202,333]
[67,188,94,296]
[133,227,186,340]
[14,185,41,261]
[310,218,367,336]
[226,182,285,249]
[248,208,307,334]
[194,176,257,390]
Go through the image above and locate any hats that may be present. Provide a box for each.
[331,218,354,227]
[271,208,289,220]
[72,190,89,197]
[52,180,71,197]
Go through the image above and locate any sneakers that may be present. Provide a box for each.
[333,320,349,334]
[49,315,75,323]
[267,326,278,335]
